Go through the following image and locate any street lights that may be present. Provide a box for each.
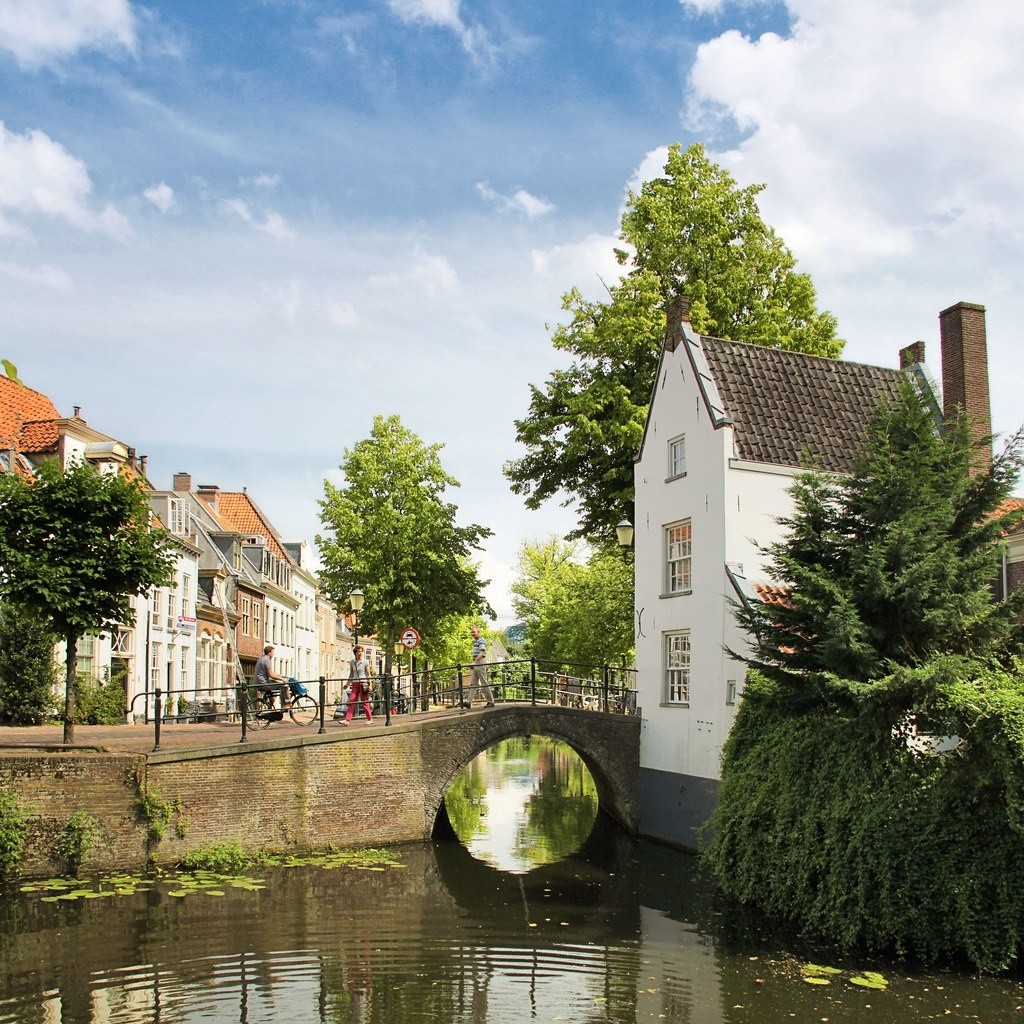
[350,581,365,717]
[394,640,404,714]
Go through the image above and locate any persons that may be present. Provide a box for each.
[253,646,292,707]
[458,627,496,708]
[339,646,373,726]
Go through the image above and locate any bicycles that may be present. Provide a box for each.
[245,676,319,732]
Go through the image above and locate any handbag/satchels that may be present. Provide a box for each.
[340,690,348,704]
[284,676,307,696]
[360,682,374,693]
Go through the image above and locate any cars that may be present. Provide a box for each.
[390,693,411,714]
[333,689,384,720]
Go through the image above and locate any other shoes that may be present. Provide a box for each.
[338,719,350,727]
[365,719,374,725]
[459,702,471,708]
[484,702,495,708]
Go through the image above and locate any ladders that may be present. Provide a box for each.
[214,585,259,727]
